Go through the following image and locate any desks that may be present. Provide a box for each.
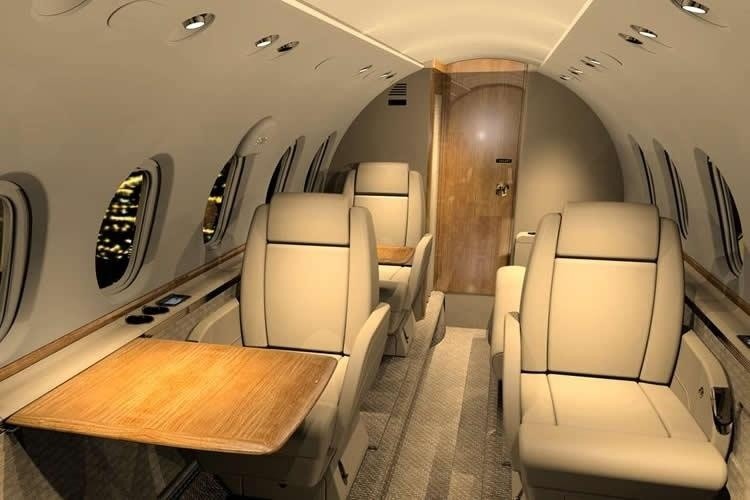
[7,336,337,499]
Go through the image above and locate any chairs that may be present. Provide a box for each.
[188,190,393,499]
[502,200,734,499]
[340,163,433,358]
